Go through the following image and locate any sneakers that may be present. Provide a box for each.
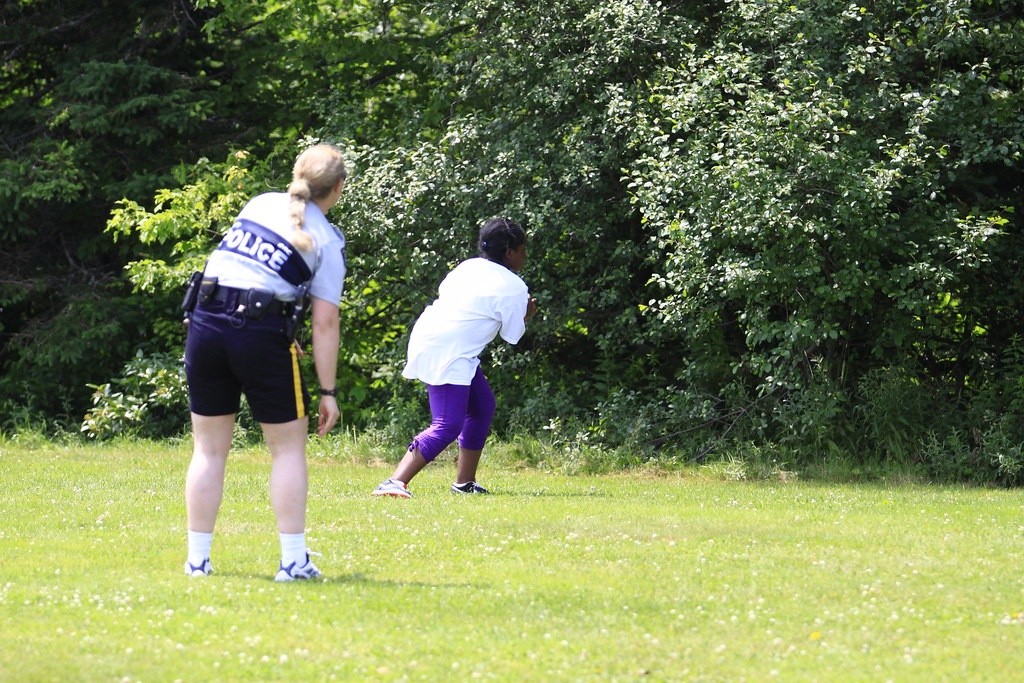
[370,478,413,498]
[451,482,490,495]
[185,558,216,576]
[275,554,323,581]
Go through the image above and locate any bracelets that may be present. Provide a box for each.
[320,388,337,396]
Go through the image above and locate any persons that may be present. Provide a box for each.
[181,143,349,585]
[370,215,538,499]
